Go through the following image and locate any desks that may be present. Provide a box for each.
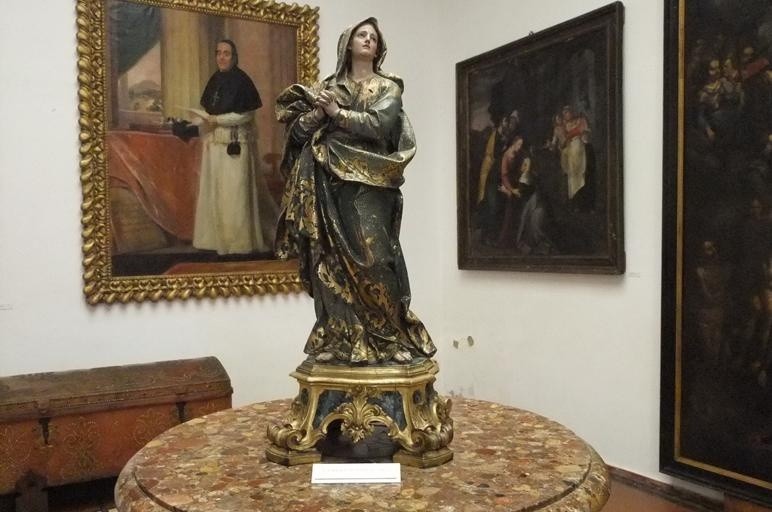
[113,387,614,512]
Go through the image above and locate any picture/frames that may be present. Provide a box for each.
[454,1,629,278]
[75,0,329,306]
[656,0,772,507]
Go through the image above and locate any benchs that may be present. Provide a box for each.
[1,352,239,501]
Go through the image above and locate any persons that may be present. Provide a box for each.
[478,104,593,252]
[686,31,770,392]
[195,39,282,255]
[273,17,437,366]
